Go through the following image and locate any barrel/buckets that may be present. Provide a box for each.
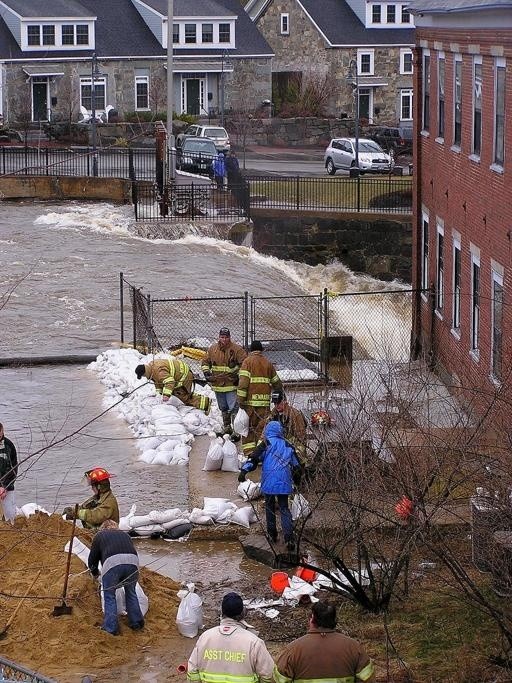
[298,567,315,581]
[271,572,290,593]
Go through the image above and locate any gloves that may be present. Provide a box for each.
[237,402,245,409]
[62,507,79,519]
[238,469,246,481]
[204,371,216,382]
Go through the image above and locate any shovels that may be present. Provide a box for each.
[53,503,79,617]
[0,569,42,640]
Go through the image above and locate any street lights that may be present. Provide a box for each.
[89,51,102,175]
[346,58,362,177]
[219,46,232,126]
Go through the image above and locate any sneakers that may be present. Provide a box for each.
[205,397,212,416]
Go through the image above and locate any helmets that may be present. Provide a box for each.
[85,468,110,482]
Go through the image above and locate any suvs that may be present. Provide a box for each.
[176,133,219,179]
[370,125,412,157]
[176,123,231,159]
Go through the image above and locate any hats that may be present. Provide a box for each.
[220,327,230,335]
[250,340,263,351]
[222,593,244,615]
[271,391,286,403]
[135,364,144,379]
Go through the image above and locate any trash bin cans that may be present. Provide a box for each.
[489,530,512,597]
[470,495,512,574]
[321,336,353,390]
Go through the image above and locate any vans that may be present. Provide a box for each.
[321,137,396,175]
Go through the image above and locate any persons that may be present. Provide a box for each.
[213,153,225,186]
[226,151,239,188]
[202,328,309,550]
[63,469,119,582]
[87,520,146,634]
[275,601,375,683]
[0,424,18,523]
[135,360,212,415]
[186,593,275,682]
